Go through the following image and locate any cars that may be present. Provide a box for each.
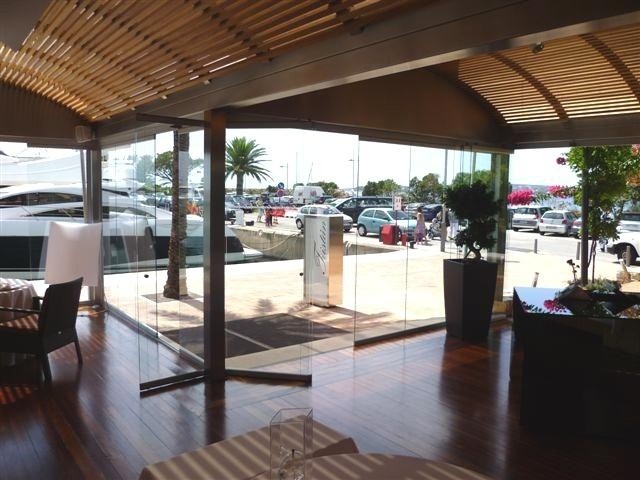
[158,185,417,280]
[572,211,640,264]
[403,198,578,238]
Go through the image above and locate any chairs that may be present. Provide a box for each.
[0,275,84,389]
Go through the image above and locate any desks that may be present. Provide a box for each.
[0,278,51,406]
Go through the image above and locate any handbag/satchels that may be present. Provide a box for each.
[439,218,450,228]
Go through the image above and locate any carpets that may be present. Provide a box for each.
[158,312,351,361]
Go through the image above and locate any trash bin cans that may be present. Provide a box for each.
[381,224,399,244]
[163,202,169,211]
[235,210,245,226]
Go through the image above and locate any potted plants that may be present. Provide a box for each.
[438,178,506,340]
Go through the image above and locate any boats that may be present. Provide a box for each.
[0,150,265,281]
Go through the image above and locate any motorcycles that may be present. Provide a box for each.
[427,220,450,242]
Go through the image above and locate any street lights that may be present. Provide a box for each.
[349,156,356,196]
[280,162,288,189]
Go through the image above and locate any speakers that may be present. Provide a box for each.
[74,125,92,144]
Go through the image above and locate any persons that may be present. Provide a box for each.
[428,218,441,235]
[437,210,442,228]
[415,206,426,245]
[255,198,274,227]
[448,209,459,240]
